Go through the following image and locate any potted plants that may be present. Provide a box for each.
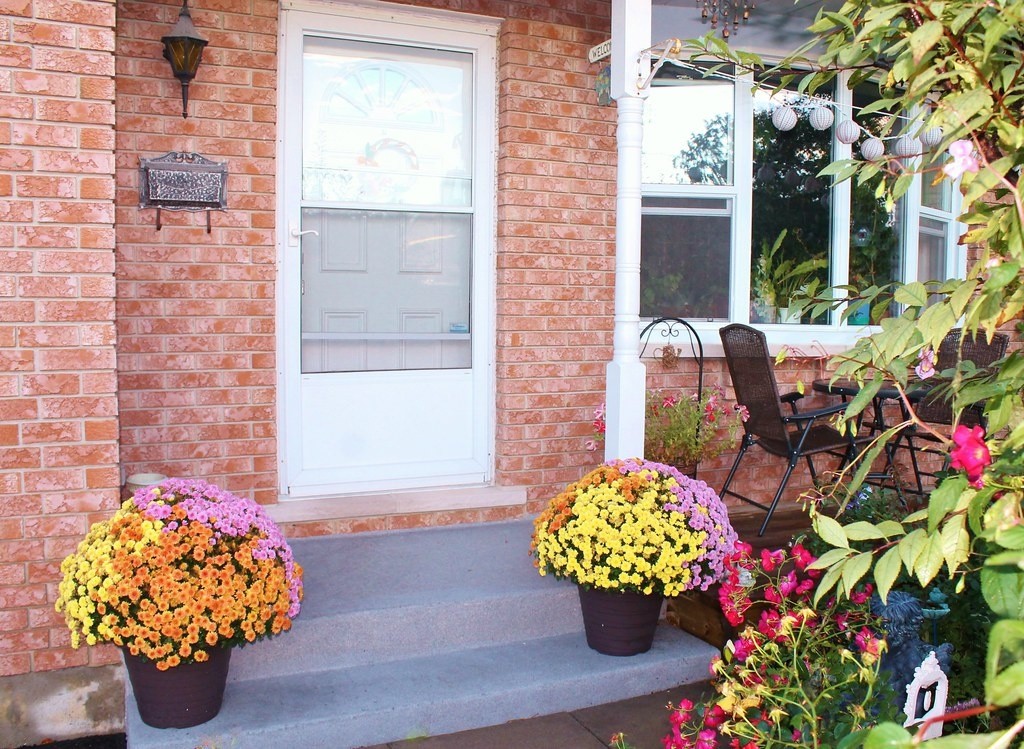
[774,252,828,322]
[754,228,788,323]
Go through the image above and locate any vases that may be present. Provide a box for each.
[667,462,698,481]
[578,586,664,656]
[848,299,871,324]
[120,647,232,729]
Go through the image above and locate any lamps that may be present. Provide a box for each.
[161,0,208,121]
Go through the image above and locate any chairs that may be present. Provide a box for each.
[718,323,877,537]
[861,328,1008,496]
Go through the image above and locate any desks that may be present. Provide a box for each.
[812,378,932,512]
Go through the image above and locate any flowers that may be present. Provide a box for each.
[55,478,303,671]
[583,383,751,465]
[528,457,744,599]
[846,271,867,299]
[661,540,889,749]
